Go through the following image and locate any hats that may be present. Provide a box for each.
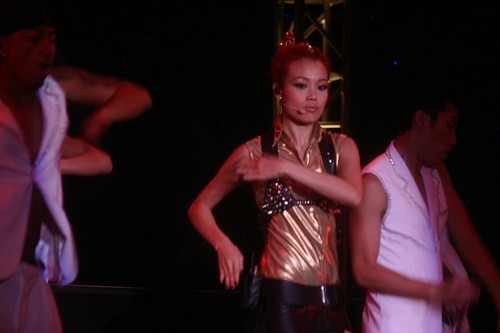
[0,0,54,38]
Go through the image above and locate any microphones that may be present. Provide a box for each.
[279,101,306,115]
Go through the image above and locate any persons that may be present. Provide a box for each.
[348,93,500,333]
[0,0,152,333]
[188,31,364,333]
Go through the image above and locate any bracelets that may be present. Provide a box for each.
[214,236,229,250]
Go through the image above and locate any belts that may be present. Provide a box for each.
[258,277,356,307]
